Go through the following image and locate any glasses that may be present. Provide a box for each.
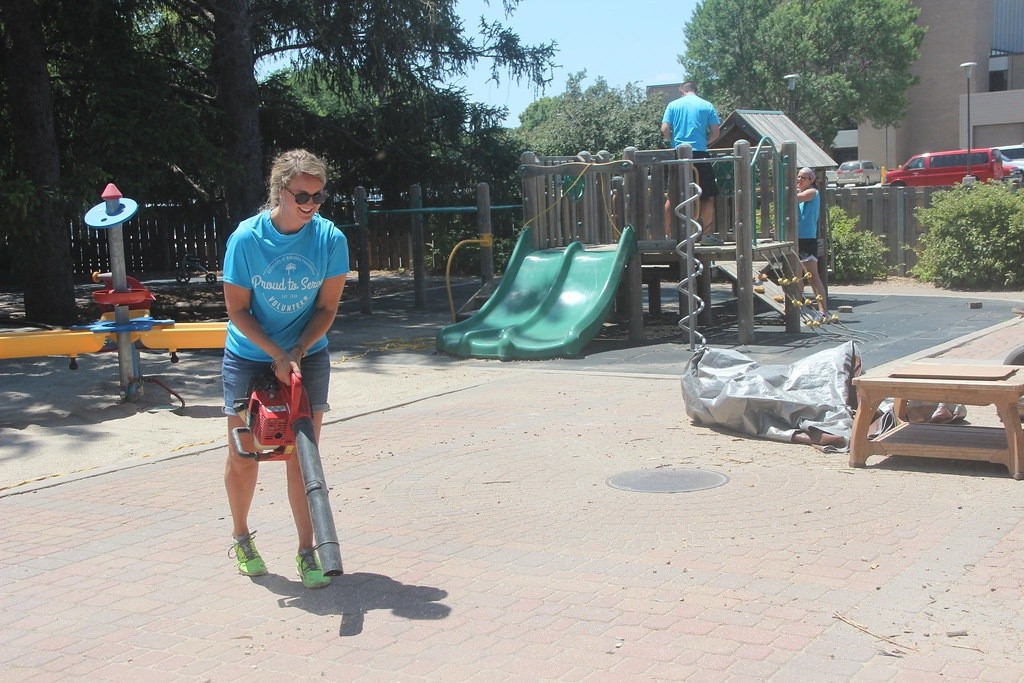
[797,176,810,181]
[285,186,330,205]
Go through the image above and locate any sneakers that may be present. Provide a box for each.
[228,529,268,576]
[700,233,724,245]
[296,545,330,587]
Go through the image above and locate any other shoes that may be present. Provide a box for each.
[814,312,827,324]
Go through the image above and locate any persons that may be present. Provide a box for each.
[220,150,349,590]
[661,82,724,246]
[796,167,830,323]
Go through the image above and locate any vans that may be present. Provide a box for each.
[881,149,1004,188]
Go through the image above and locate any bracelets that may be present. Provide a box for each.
[291,344,308,358]
[272,351,289,365]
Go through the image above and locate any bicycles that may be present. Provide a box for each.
[175,251,217,285]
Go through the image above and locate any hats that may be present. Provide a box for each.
[800,167,815,183]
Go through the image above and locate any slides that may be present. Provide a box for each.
[435,223,637,361]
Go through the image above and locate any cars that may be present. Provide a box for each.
[825,165,838,185]
[989,145,1024,187]
[837,160,881,189]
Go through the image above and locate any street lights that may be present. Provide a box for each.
[784,74,802,121]
[958,61,978,185]
